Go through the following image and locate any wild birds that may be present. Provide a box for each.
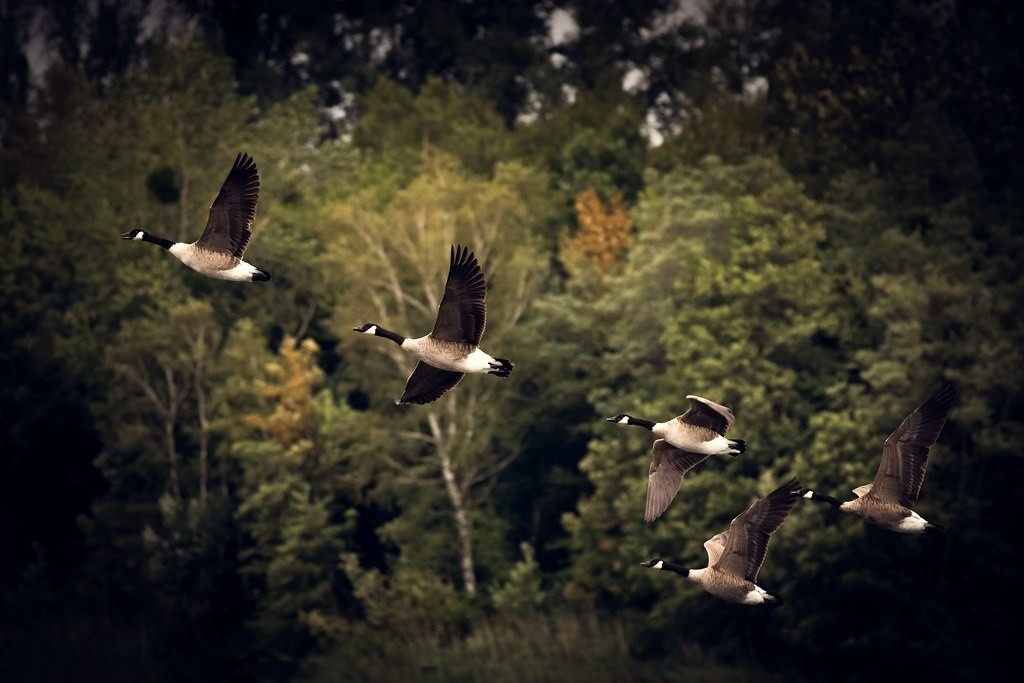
[353,243,516,406]
[640,476,802,612]
[120,151,272,283]
[606,394,747,522]
[790,379,958,537]
